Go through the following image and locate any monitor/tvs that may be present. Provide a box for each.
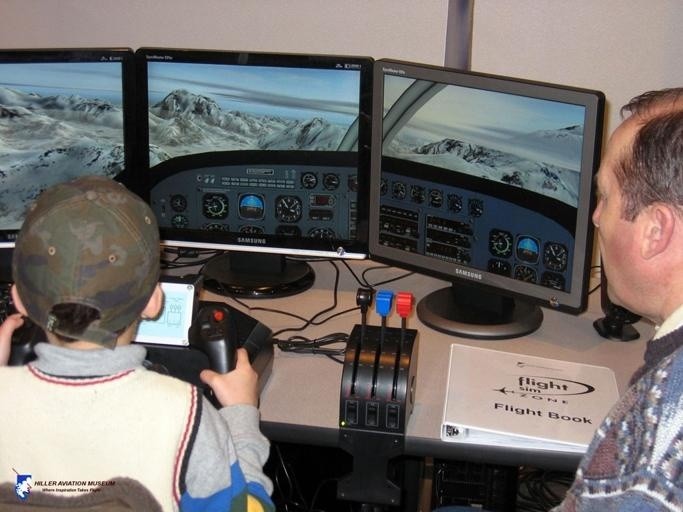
[1,46,132,254]
[369,58,605,339]
[134,47,370,297]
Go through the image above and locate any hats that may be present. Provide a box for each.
[12,175,163,351]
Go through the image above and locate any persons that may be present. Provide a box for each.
[549,87,682,512]
[0,174,278,512]
